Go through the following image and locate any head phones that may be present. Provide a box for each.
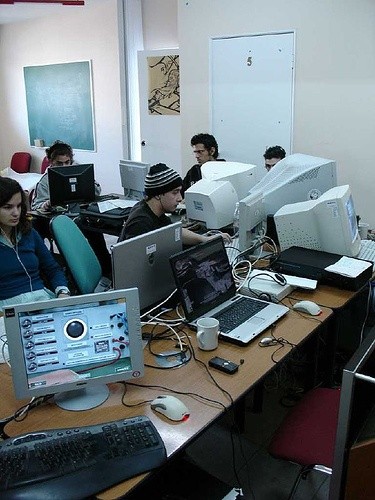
[248,273,287,302]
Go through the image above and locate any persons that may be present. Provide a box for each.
[263,145,286,172]
[180,133,226,204]
[117,163,233,245]
[0,177,70,316]
[31,140,112,282]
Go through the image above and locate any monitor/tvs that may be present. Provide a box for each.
[3,153,363,411]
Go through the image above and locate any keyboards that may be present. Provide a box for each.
[357,240,375,264]
[220,239,240,266]
[0,415,168,500]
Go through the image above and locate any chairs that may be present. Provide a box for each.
[268,386,341,500]
[11,152,53,174]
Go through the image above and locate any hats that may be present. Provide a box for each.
[144,163,182,196]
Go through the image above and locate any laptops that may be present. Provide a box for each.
[168,234,290,346]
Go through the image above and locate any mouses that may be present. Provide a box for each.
[292,300,322,316]
[150,395,190,421]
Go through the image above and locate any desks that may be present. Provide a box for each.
[0,246,374,500]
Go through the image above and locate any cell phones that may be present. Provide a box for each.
[209,355,238,375]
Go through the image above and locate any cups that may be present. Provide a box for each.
[358,222,369,239]
[196,317,220,350]
